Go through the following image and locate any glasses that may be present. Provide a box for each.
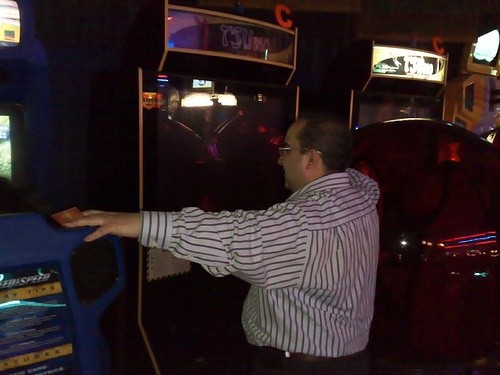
[279,147,327,160]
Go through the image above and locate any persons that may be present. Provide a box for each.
[64,114,381,375]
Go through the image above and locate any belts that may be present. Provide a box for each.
[253,346,340,370]
